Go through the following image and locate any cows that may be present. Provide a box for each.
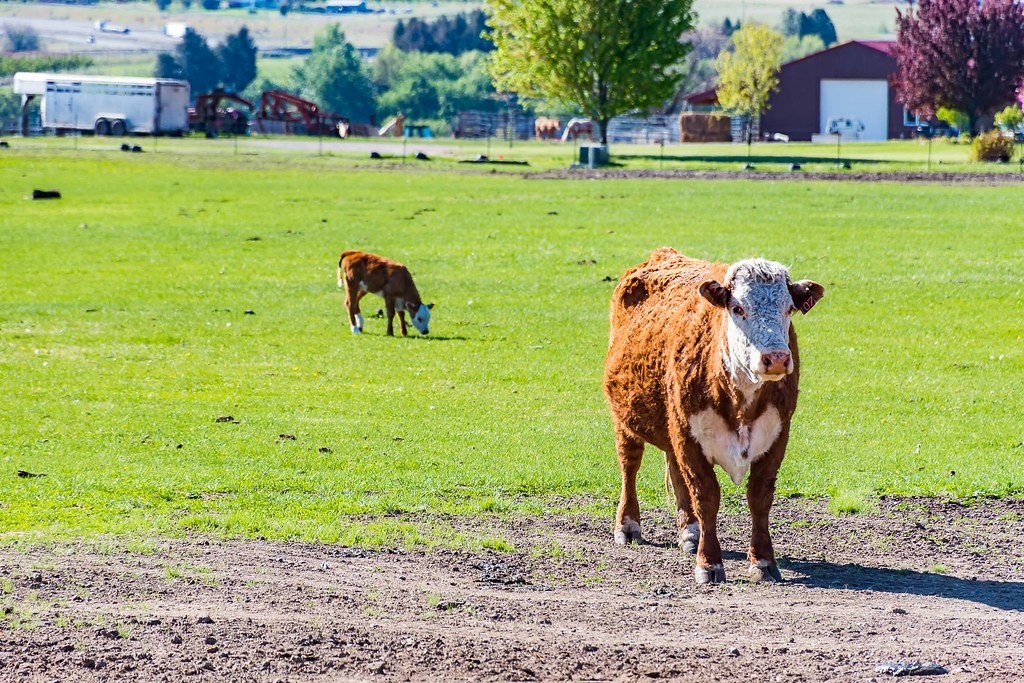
[336,249,434,339]
[602,246,825,586]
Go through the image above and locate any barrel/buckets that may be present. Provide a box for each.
[580,145,609,170]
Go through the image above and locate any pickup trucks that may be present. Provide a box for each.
[450,111,493,137]
[912,120,959,138]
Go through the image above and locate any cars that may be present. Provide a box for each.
[1013,125,1024,143]
